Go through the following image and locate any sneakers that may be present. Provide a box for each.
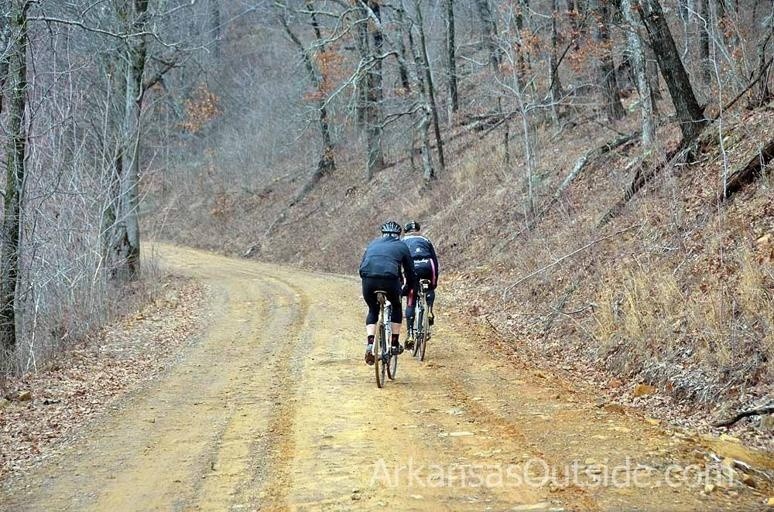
[364,350,375,365]
[391,343,403,355]
[428,312,434,325]
[404,338,415,350]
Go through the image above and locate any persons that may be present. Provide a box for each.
[357,221,416,365]
[400,221,439,351]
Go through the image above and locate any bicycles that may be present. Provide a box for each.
[403,278,435,361]
[369,288,400,389]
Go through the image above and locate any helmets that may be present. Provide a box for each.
[381,221,401,235]
[404,221,421,234]
